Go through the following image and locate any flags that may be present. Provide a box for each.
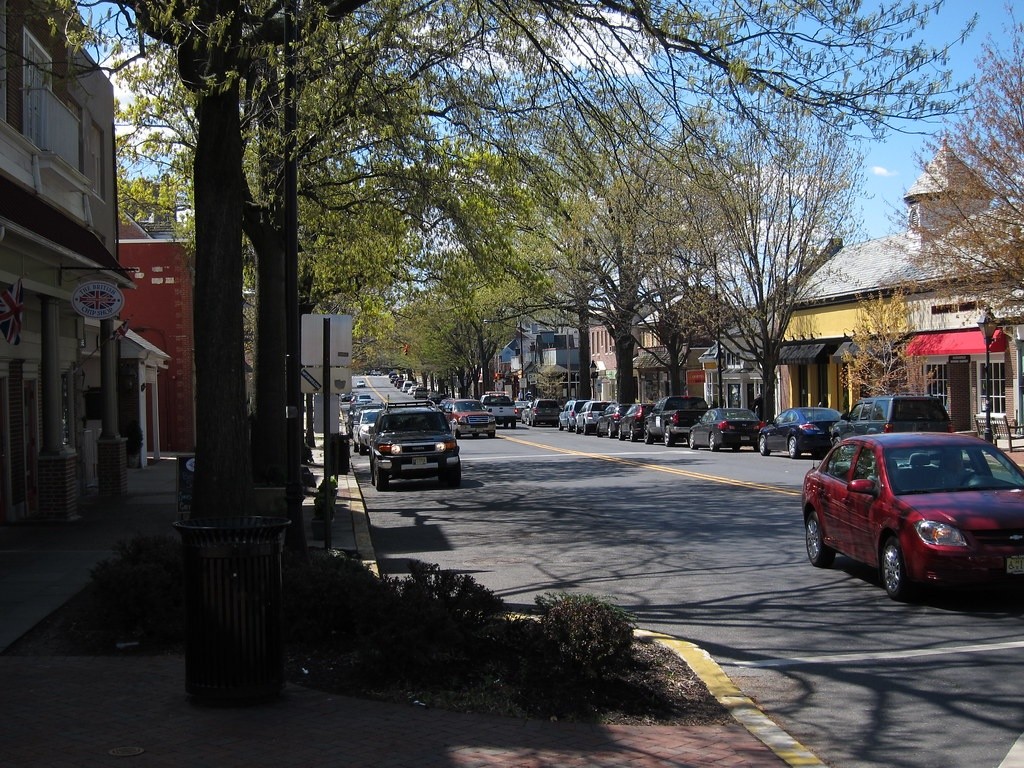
[0,279,24,346]
[111,320,128,340]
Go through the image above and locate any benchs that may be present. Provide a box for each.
[973,414,1024,453]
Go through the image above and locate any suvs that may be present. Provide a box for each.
[439,397,496,438]
[575,401,616,435]
[557,399,591,432]
[368,400,461,492]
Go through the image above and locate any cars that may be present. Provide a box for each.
[514,401,529,419]
[801,432,1024,601]
[388,371,448,405]
[520,402,534,425]
[617,403,657,441]
[338,391,385,456]
[595,403,633,438]
[526,398,562,427]
[356,381,365,388]
[688,407,766,452]
[370,370,382,376]
[758,407,844,460]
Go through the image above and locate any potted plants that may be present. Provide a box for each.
[309,475,339,540]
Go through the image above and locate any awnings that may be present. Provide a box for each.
[905,329,1006,356]
[773,344,829,364]
[832,339,901,363]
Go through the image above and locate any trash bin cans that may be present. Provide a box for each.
[173,513,292,708]
[331,433,350,475]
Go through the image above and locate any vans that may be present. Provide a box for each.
[829,395,952,459]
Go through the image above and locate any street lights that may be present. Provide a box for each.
[976,307,997,446]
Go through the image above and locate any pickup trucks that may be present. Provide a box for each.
[479,393,518,429]
[643,396,710,449]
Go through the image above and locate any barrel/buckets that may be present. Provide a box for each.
[174,516,292,707]
